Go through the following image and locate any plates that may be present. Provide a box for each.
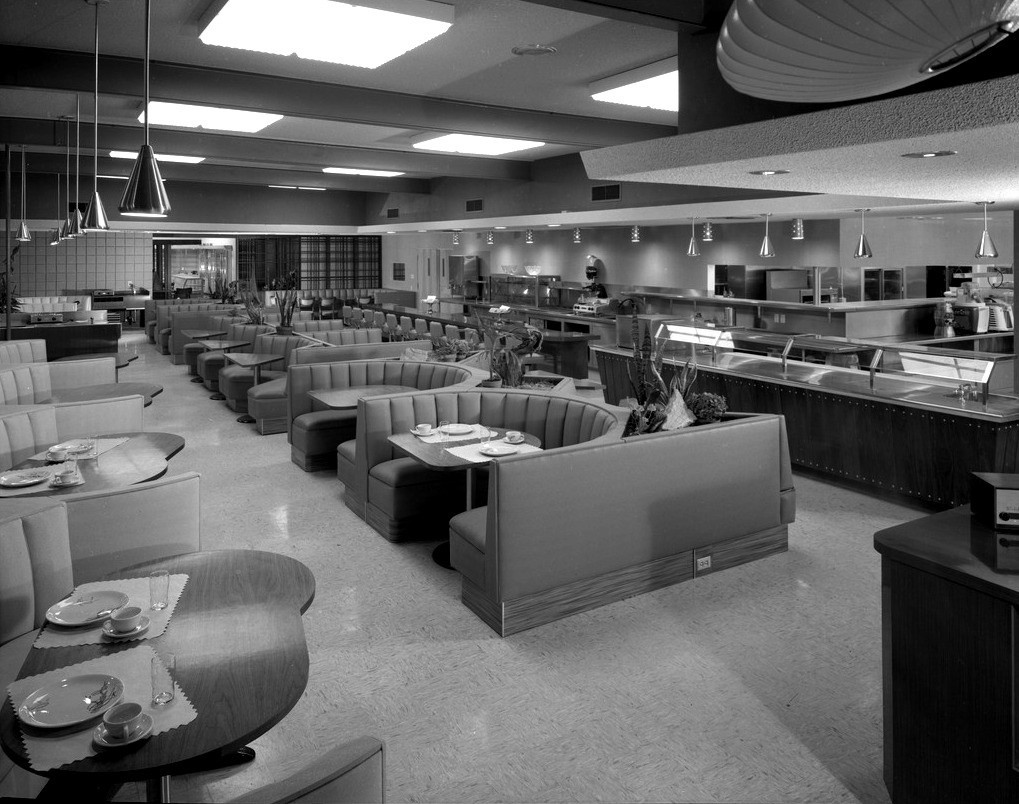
[47,456,69,461]
[93,713,154,747]
[49,442,91,452]
[18,673,124,728]
[0,468,53,486]
[480,437,524,455]
[103,616,151,638]
[51,479,79,486]
[46,590,130,625]
[410,424,473,435]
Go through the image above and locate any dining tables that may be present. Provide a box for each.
[36,382,164,409]
[386,426,541,571]
[224,353,285,423]
[199,339,251,401]
[181,329,228,383]
[0,433,185,495]
[2,548,343,804]
[306,384,421,442]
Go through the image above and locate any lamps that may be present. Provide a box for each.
[12,0,1019,261]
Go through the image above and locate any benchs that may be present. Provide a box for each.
[0,289,794,803]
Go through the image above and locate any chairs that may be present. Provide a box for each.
[356,296,373,309]
[314,296,336,320]
[298,298,314,314]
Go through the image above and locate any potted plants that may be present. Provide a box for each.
[472,310,529,391]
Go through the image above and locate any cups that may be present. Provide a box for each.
[480,426,490,443]
[65,453,77,475]
[111,607,142,631]
[506,431,524,442]
[440,421,450,441]
[46,446,67,458]
[150,570,169,610]
[55,471,74,483]
[416,424,432,434]
[152,653,175,705]
[88,436,98,455]
[104,703,142,740]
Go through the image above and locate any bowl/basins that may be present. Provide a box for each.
[502,265,522,275]
[524,265,543,275]
